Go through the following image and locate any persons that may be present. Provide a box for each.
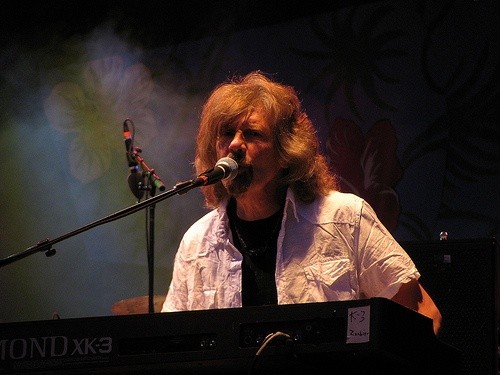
[158,70,443,338]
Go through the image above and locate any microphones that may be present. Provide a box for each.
[122,121,145,199]
[192,157,239,186]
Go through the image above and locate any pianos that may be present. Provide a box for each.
[0,297,438,375]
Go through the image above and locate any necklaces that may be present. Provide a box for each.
[231,216,282,258]
